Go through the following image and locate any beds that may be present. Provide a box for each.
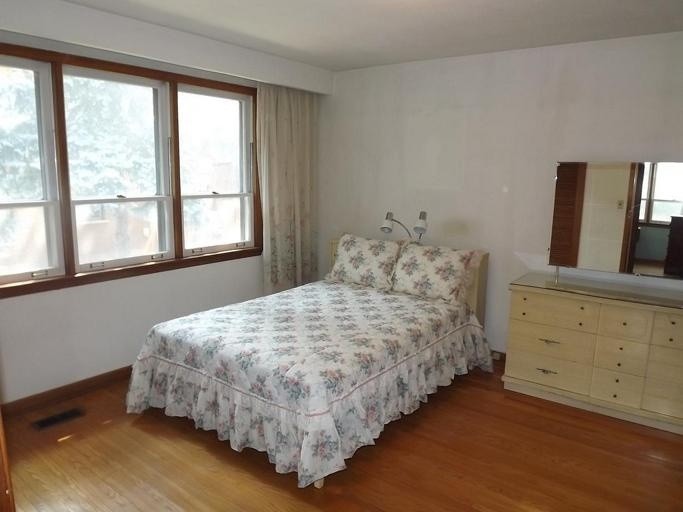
[124,238,492,490]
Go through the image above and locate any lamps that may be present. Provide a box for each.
[379,210,429,243]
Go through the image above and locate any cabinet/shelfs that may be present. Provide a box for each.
[500,268,683,437]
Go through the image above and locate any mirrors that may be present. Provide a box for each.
[545,160,683,281]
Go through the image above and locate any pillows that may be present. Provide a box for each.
[324,232,478,310]
